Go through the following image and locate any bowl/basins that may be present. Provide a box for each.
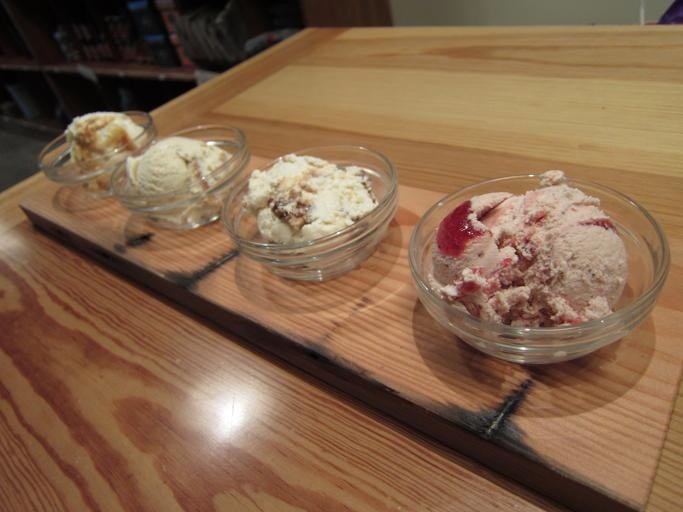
[35,109,159,201]
[109,121,254,231]
[222,139,401,283]
[407,171,673,369]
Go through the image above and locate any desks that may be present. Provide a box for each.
[0,22,682,511]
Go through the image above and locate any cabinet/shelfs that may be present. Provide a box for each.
[0,0,393,144]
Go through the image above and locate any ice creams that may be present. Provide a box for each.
[243,150,379,247]
[427,167,628,336]
[64,112,146,190]
[126,135,232,204]
